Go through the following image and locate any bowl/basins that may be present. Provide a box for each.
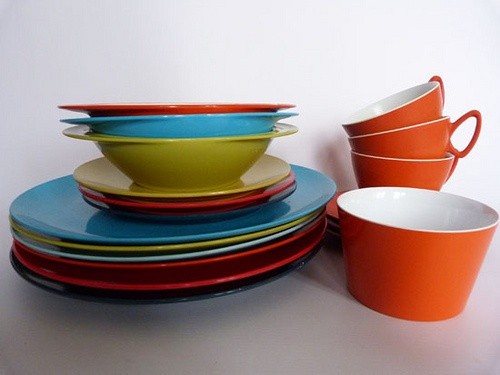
[58,104,299,191]
[336,187,500,321]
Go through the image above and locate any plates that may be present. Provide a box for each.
[9,156,350,305]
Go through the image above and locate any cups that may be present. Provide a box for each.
[348,110,482,159]
[351,151,459,191]
[342,75,445,137]
